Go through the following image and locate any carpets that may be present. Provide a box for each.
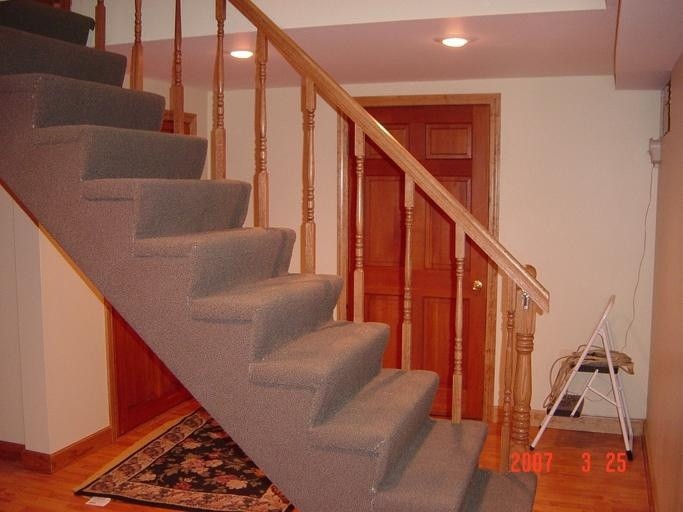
[73,402,297,511]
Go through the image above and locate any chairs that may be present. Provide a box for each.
[529,295,636,464]
